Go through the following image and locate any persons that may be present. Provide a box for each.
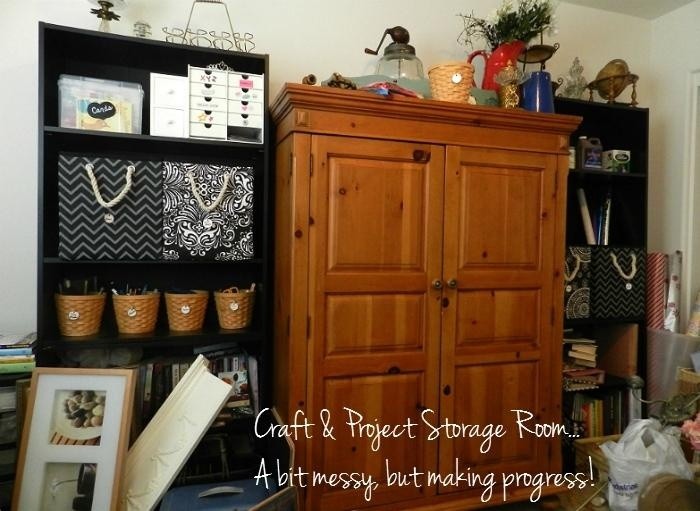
[59,343,141,369]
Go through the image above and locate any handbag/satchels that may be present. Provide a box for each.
[592,244,645,318]
[57,154,164,262]
[564,246,591,319]
[162,160,256,262]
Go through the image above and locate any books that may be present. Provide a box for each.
[134,340,259,429]
[563,338,643,440]
[0,328,38,375]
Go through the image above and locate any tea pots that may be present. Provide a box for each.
[467,38,528,92]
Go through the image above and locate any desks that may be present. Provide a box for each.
[572,433,625,511]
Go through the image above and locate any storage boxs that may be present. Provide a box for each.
[56,74,146,134]
[185,62,267,146]
[150,73,189,140]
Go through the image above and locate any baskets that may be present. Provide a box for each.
[165,289,209,331]
[428,60,475,104]
[54,290,107,337]
[213,286,254,330]
[679,366,700,395]
[112,292,159,334]
[680,432,700,464]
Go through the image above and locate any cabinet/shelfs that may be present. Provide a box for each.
[37,20,275,487]
[273,82,584,510]
[553,95,648,472]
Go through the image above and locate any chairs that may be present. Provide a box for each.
[159,408,298,511]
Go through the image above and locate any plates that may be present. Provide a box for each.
[54,390,104,439]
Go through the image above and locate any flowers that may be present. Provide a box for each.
[454,0,559,59]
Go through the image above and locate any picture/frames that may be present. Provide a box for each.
[11,367,138,510]
